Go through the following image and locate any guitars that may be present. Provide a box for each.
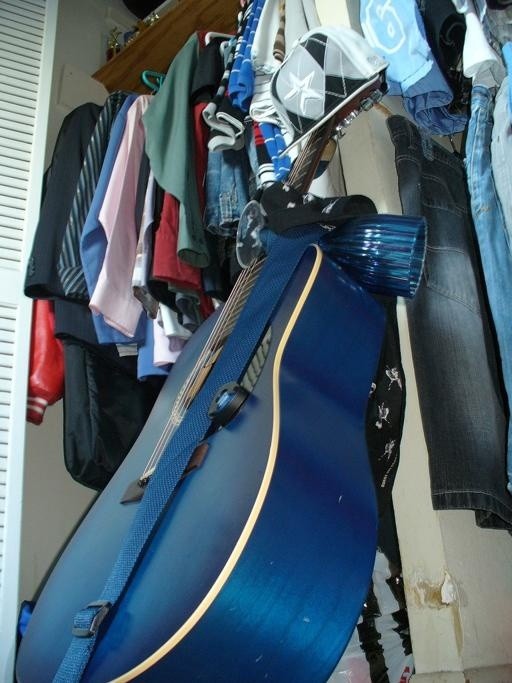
[15,71,389,682]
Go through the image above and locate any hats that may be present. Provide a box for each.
[270,26,390,158]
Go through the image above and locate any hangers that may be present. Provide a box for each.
[141,69,166,92]
[204,31,236,46]
[219,39,229,57]
